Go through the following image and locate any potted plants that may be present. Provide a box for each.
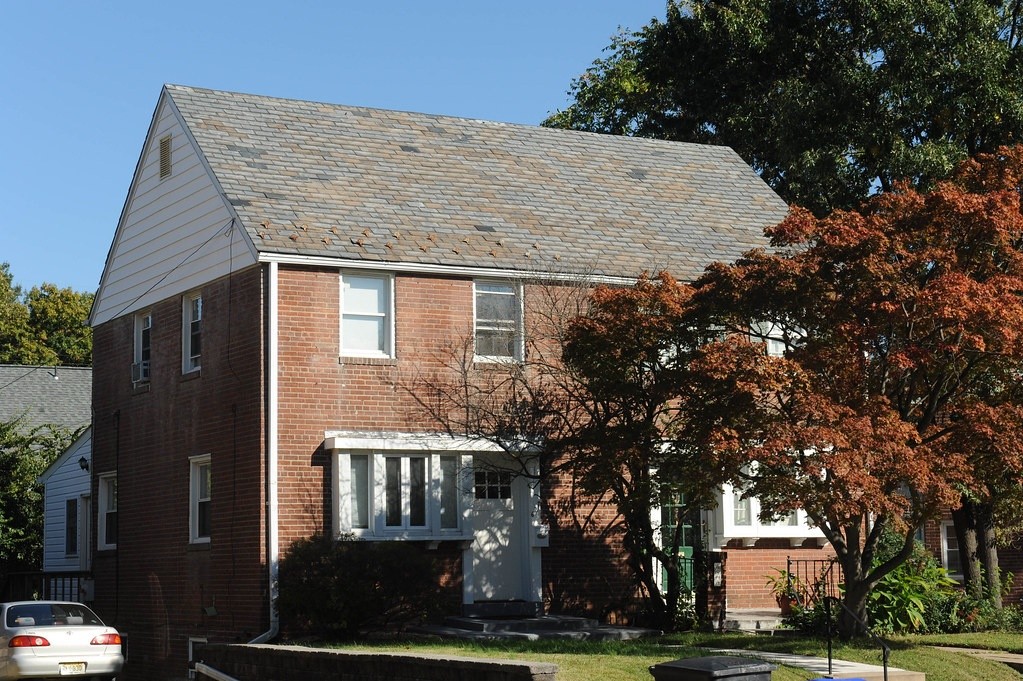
[762,565,806,618]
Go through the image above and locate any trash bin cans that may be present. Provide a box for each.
[648,655,773,681]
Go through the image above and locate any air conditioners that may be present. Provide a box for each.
[130,361,150,383]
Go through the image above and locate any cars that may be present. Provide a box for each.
[0,600,125,681]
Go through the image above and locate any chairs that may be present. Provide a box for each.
[66,616,83,625]
[17,617,35,626]
